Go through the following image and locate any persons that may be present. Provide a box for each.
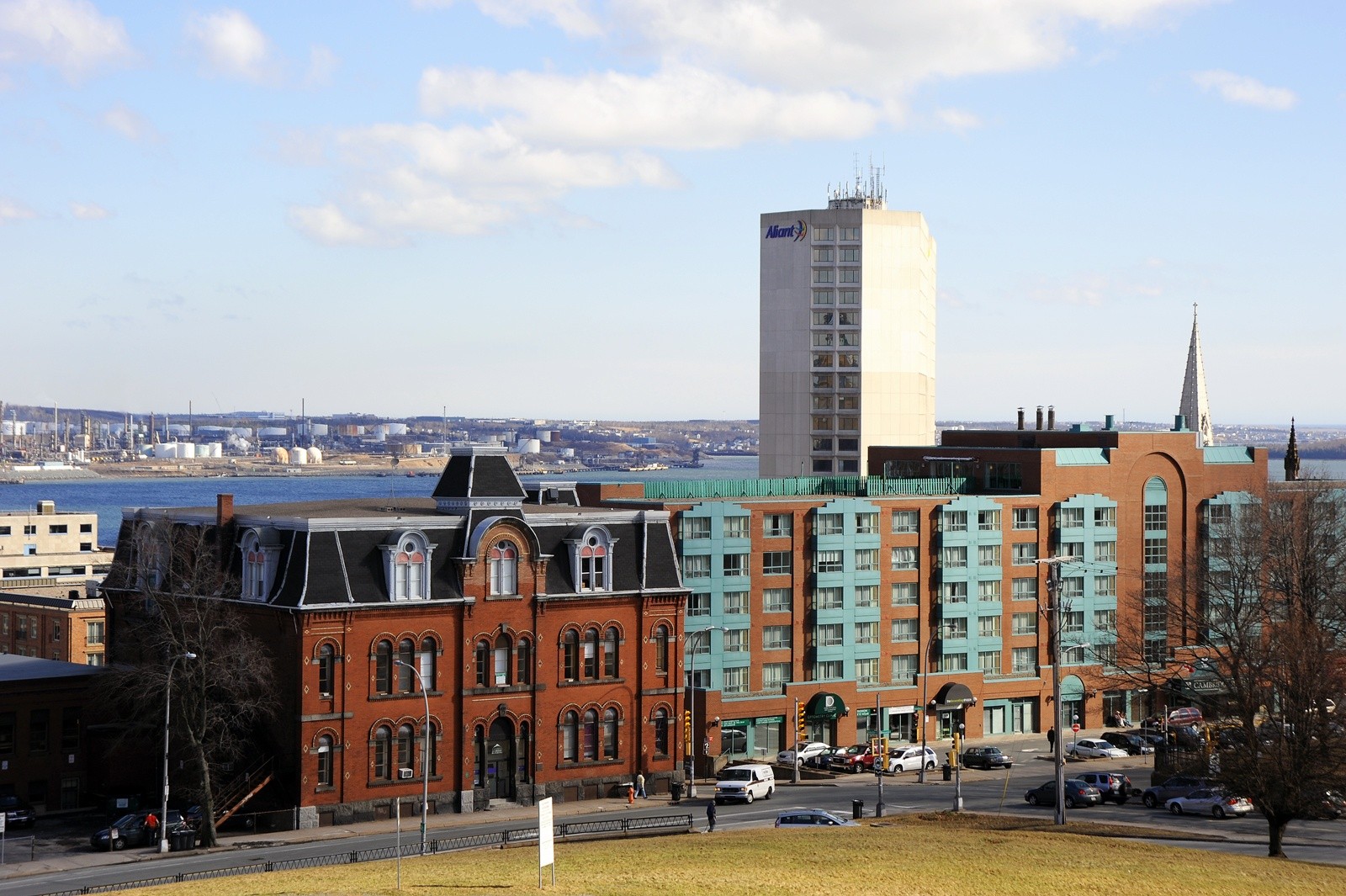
[634,771,648,799]
[706,800,716,832]
[1047,726,1054,752]
[1113,709,1133,728]
[174,815,186,831]
[143,811,160,847]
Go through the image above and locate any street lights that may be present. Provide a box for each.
[1073,714,1078,760]
[158,652,196,852]
[395,660,430,855]
[688,628,730,798]
[959,723,965,770]
[1059,642,1091,766]
[918,624,956,782]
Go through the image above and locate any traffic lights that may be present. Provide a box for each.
[909,710,923,743]
[798,702,806,731]
[951,732,959,749]
[798,733,808,741]
[945,750,957,767]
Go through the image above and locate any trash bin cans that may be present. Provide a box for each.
[853,799,863,819]
[943,764,951,781]
[672,782,681,800]
[171,830,196,851]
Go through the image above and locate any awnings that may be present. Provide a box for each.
[923,456,978,462]
[937,681,973,703]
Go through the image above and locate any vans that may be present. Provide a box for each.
[715,763,775,803]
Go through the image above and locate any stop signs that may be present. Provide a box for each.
[1071,723,1081,732]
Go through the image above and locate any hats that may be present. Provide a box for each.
[1116,711,1120,714]
[712,800,716,804]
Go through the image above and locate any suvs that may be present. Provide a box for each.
[1302,698,1337,715]
[90,810,187,851]
[1140,708,1203,732]
[1074,772,1133,806]
[1142,776,1224,809]
[774,809,863,828]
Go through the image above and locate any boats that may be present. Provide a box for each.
[618,451,669,471]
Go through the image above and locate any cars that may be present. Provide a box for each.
[1024,779,1102,808]
[1164,787,1255,819]
[1299,788,1346,819]
[1065,738,1130,760]
[1100,726,1205,754]
[958,746,1014,770]
[721,729,747,752]
[1140,711,1171,728]
[778,742,939,773]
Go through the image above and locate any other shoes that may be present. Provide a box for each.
[1122,726,1125,728]
[634,798,637,799]
[708,830,710,832]
[644,796,648,799]
[711,830,713,832]
[1131,725,1134,728]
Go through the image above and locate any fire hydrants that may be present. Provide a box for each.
[627,787,634,803]
